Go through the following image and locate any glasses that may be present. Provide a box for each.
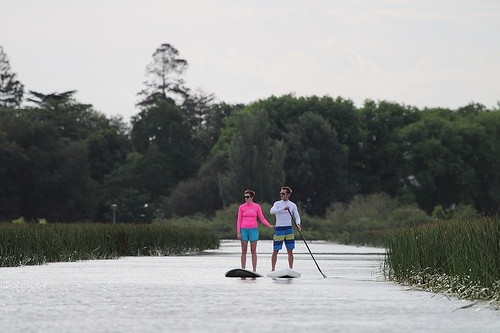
[243,196,253,198]
[279,192,287,195]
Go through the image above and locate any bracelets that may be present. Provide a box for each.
[298,224,300,225]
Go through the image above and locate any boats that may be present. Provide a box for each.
[224,267,263,278]
[267,268,301,279]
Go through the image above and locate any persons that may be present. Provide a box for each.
[269,186,301,272]
[236,189,275,272]
